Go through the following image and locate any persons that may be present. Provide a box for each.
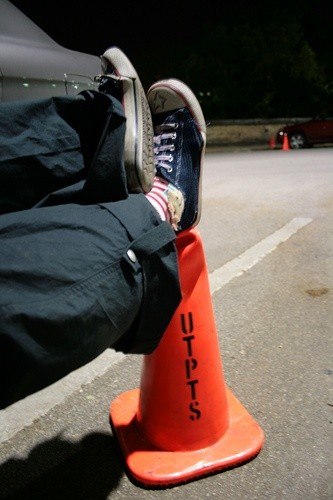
[1,46,207,411]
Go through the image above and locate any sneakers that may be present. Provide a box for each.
[145,78,207,235]
[98,47,155,193]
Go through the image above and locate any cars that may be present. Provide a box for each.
[278,112,333,149]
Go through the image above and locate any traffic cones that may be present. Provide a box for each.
[281,133,290,151]
[270,135,276,149]
[106,232,267,487]
